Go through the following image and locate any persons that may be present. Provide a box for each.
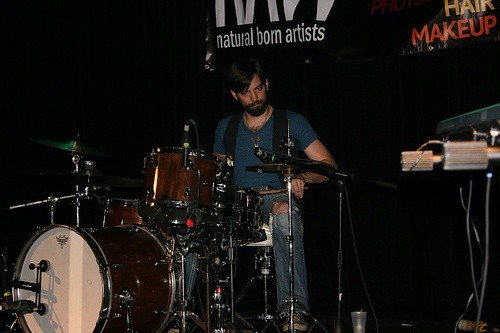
[185,56,339,331]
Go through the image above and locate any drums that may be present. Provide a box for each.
[104,197,141,227]
[138,147,227,226]
[11,224,187,333]
[212,184,265,244]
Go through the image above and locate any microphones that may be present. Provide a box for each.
[181,121,191,147]
[253,148,276,164]
[0,300,34,314]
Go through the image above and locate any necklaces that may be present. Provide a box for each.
[245,106,270,147]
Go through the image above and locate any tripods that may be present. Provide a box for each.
[159,164,328,333]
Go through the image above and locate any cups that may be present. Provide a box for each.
[350,311,367,333]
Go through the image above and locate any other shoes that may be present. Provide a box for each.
[283,315,308,331]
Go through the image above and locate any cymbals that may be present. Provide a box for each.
[32,138,116,158]
[243,163,335,172]
[28,170,134,178]
